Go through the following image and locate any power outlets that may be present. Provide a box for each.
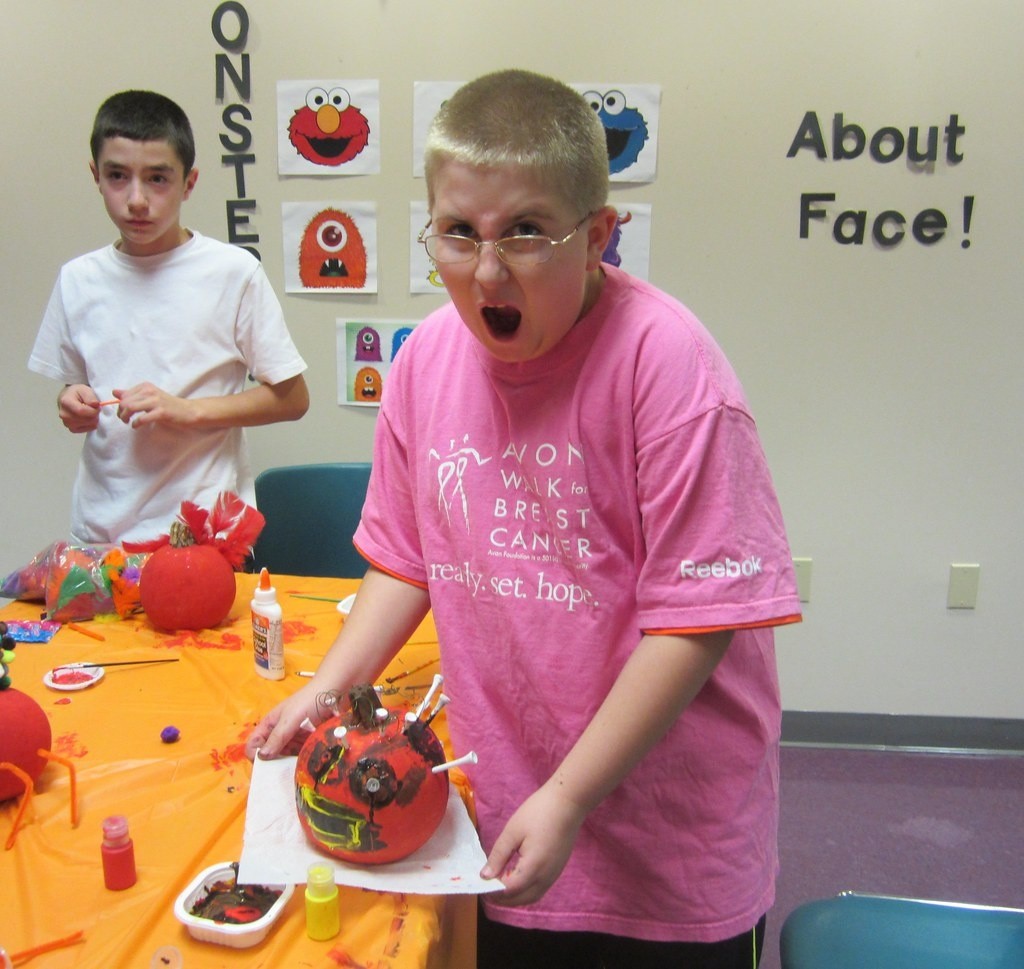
[792,557,813,603]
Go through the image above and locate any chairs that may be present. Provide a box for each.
[246,463,372,579]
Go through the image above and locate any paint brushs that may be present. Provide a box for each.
[386,658,440,684]
[53,657,180,672]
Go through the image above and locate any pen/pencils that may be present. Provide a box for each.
[98,398,120,406]
[295,671,383,691]
[67,621,106,643]
[289,594,341,603]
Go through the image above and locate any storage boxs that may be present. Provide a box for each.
[777,888,1024,969]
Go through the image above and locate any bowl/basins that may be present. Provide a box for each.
[337,594,356,622]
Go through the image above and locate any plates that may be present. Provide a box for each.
[43,662,104,690]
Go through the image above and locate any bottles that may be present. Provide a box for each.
[250,568,285,680]
[101,814,138,891]
[304,863,339,942]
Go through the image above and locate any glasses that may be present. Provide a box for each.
[417,213,593,266]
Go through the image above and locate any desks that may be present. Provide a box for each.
[0,571,478,969]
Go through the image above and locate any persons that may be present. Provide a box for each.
[28,89,310,548]
[245,70,804,969]
